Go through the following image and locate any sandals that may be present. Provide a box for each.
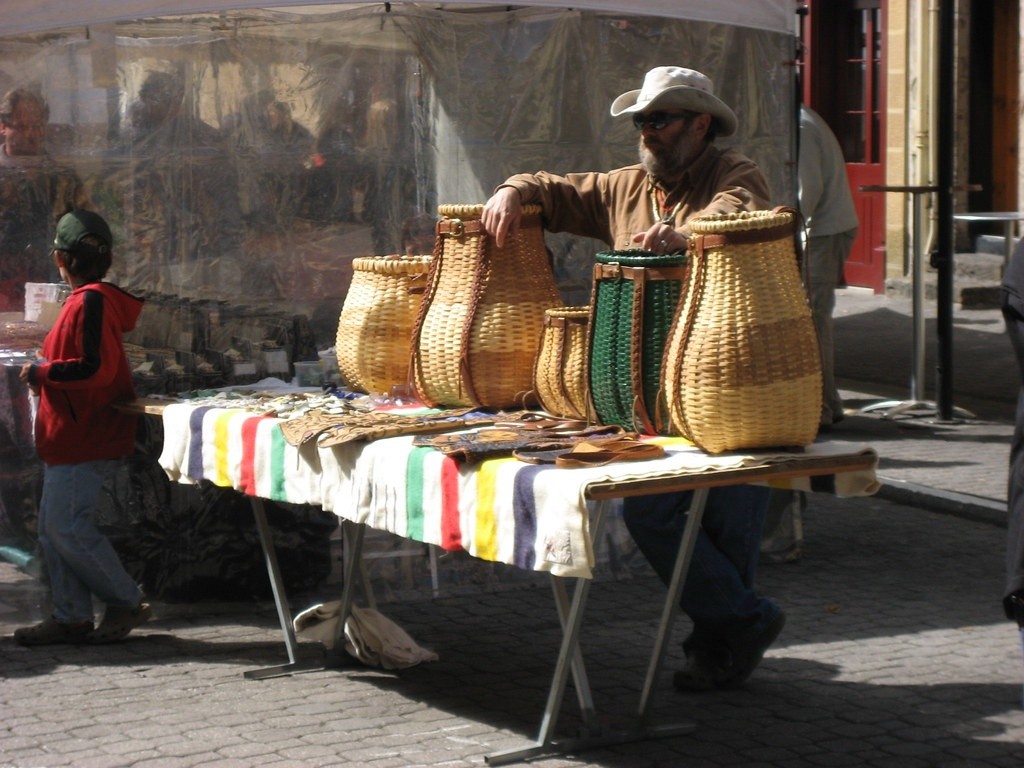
[16,602,152,646]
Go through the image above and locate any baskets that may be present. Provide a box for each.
[583,249,688,435]
[533,305,601,425]
[659,209,823,455]
[408,202,564,410]
[335,254,434,398]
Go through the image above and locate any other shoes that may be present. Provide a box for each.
[671,606,786,693]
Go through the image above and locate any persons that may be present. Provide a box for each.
[998,238,1024,629]
[798,104,859,433]
[480,66,787,693]
[14,209,154,647]
[0,87,90,298]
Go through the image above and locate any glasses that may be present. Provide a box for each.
[632,110,692,130]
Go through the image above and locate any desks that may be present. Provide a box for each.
[859,183,983,423]
[0,311,220,542]
[950,212,1024,270]
[135,384,879,768]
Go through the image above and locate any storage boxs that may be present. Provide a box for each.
[24,282,69,323]
[293,361,345,387]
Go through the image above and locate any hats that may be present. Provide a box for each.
[609,66,738,140]
[49,209,113,256]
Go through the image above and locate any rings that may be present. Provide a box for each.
[660,240,668,247]
